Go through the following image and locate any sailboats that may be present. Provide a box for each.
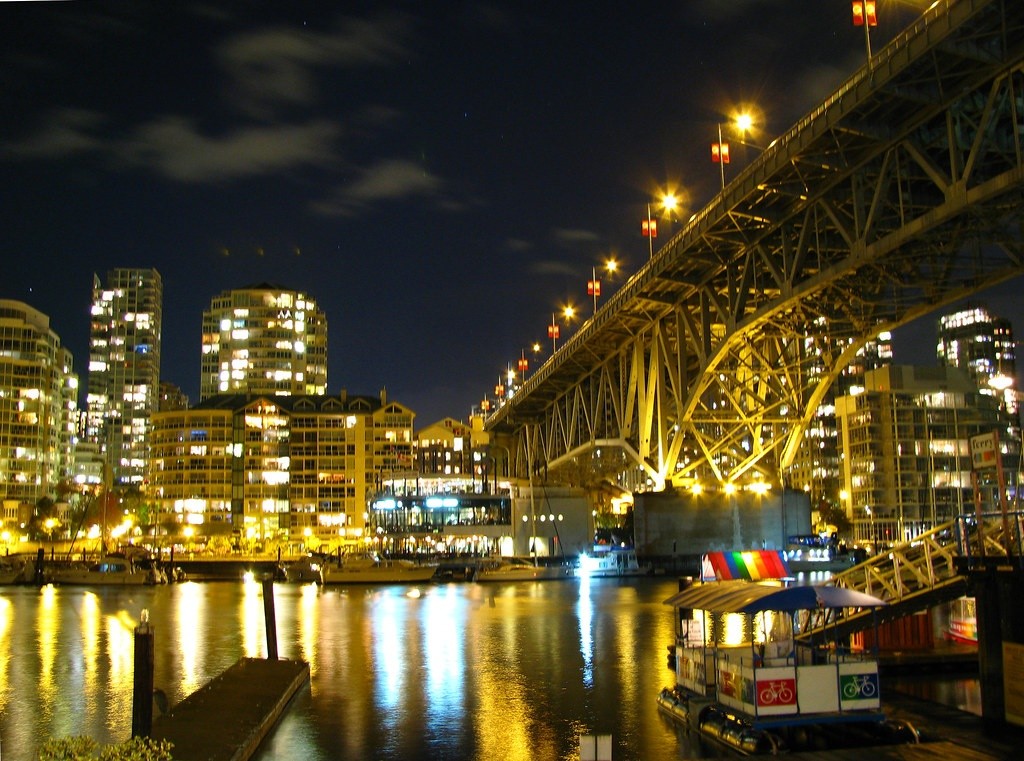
[472,424,576,582]
[50,451,153,584]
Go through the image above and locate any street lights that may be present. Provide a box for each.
[499,370,516,409]
[718,111,755,190]
[522,344,540,384]
[648,194,676,260]
[552,307,574,354]
[593,260,617,314]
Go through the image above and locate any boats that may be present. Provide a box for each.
[655,577,922,761]
[276,550,441,585]
